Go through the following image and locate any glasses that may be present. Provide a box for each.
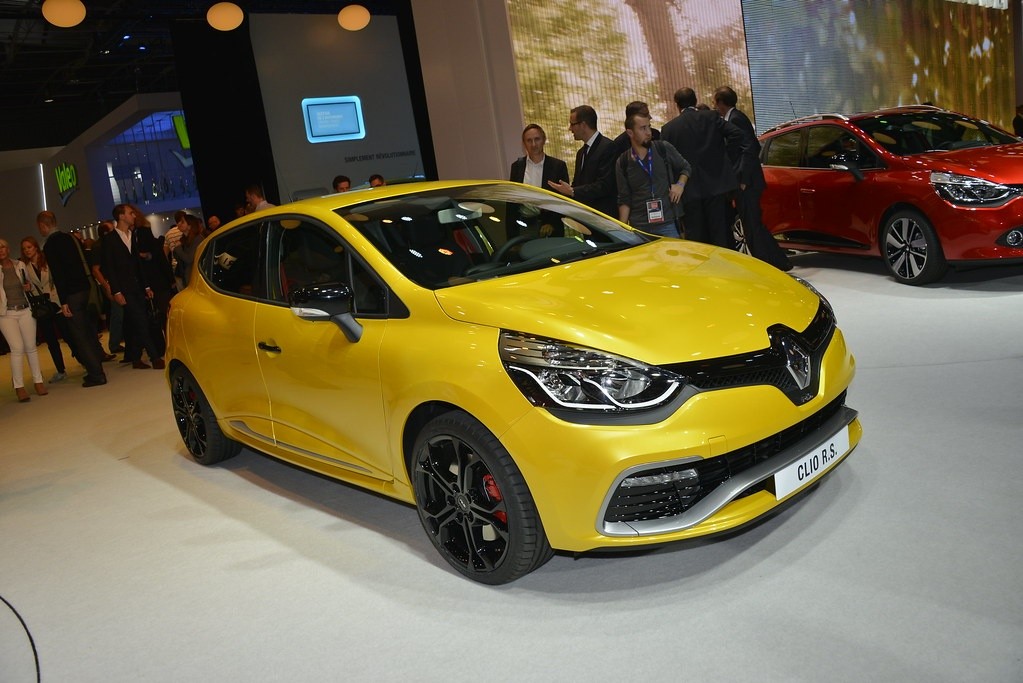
[571,120,584,127]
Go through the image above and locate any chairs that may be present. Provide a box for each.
[288,216,470,306]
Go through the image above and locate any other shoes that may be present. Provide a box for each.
[49,372,67,383]
[152,358,165,369]
[111,345,124,353]
[102,351,116,362]
[82,375,107,387]
[132,360,151,369]
[119,358,141,362]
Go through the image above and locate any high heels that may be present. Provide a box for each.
[16,387,30,401]
[35,383,48,396]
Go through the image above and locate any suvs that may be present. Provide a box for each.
[757,105,1023,286]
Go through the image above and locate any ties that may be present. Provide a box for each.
[583,144,588,158]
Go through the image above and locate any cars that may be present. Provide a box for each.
[164,180,863,585]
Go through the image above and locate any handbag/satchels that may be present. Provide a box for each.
[25,282,61,321]
[87,285,103,321]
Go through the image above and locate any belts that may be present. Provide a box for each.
[7,303,28,311]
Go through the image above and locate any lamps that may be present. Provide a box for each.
[42,0,86,28]
[338,5,371,31]
[207,1,244,31]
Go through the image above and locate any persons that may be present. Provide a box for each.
[0,239,48,401]
[72,204,245,369]
[333,176,351,193]
[37,211,107,387]
[613,101,660,152]
[649,202,661,212]
[1013,105,1023,138]
[712,85,793,272]
[548,105,622,243]
[369,175,386,187]
[659,87,745,248]
[244,183,275,212]
[505,124,569,241]
[615,113,691,238]
[17,236,86,383]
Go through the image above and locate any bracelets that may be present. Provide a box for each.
[676,181,684,187]
